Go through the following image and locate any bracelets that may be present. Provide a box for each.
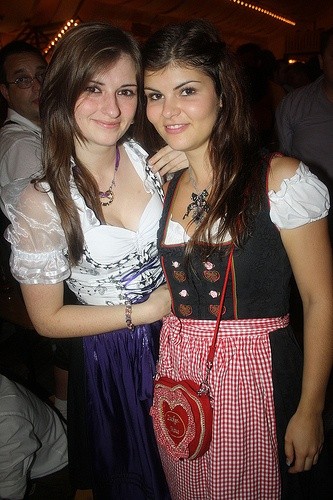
[124,304,135,332]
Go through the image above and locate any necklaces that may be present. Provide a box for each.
[68,140,121,207]
[181,160,219,221]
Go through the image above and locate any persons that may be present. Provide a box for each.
[0,21,193,500]
[135,19,333,500]
[263,41,324,100]
[272,27,333,355]
[1,38,72,423]
[0,373,74,500]
[232,43,286,143]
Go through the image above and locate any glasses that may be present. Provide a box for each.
[7,71,44,89]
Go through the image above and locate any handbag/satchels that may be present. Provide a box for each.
[149,374,214,462]
